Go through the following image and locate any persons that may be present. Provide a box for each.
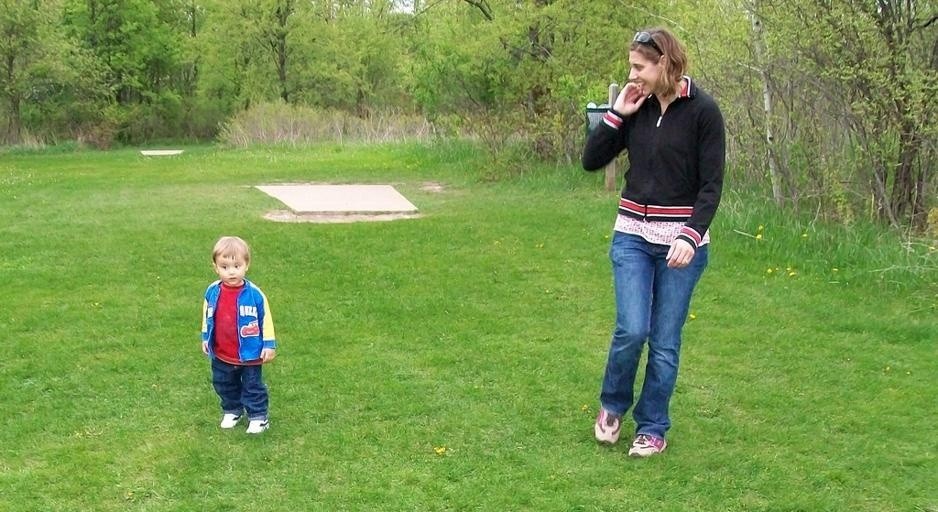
[581,25,729,462]
[200,236,277,434]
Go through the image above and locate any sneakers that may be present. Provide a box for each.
[628,433,667,458]
[220,413,242,429]
[247,420,268,434]
[595,405,623,445]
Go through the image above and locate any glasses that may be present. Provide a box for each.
[633,31,661,52]
